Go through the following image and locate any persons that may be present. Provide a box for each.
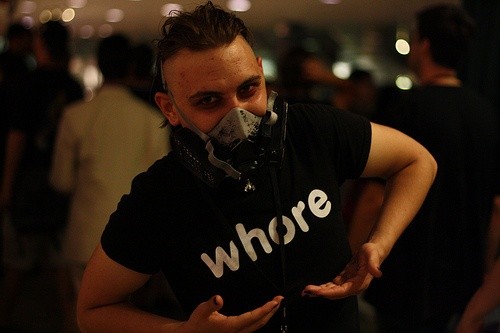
[76,1,437,333]
